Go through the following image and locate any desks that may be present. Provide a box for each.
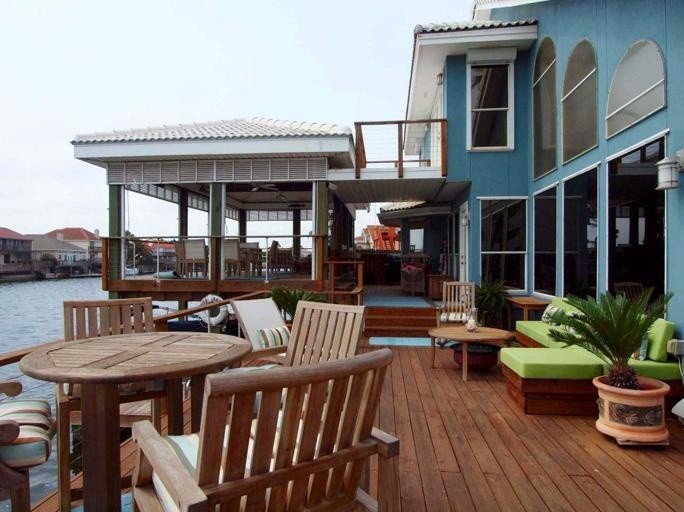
[504,297,549,330]
[428,326,514,382]
[18,330,254,511]
[399,253,430,297]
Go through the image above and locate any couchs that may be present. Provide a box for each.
[499,295,684,416]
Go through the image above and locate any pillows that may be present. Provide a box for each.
[636,332,649,360]
[561,311,596,339]
[254,324,292,350]
[541,304,565,326]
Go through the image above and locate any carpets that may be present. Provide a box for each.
[363,295,433,310]
[368,336,459,347]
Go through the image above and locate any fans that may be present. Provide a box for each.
[249,182,278,191]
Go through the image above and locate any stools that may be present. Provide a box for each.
[426,274,455,301]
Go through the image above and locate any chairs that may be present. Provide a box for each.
[131,347,401,512]
[173,238,263,279]
[229,296,348,415]
[270,241,297,274]
[56,296,184,512]
[0,379,57,512]
[435,281,479,350]
[227,300,367,419]
[613,280,648,313]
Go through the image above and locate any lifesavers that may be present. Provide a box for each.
[198,294,228,325]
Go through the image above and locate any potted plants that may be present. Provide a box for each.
[475,274,512,329]
[541,286,675,447]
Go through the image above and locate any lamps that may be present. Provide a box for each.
[461,212,469,226]
[655,156,684,191]
